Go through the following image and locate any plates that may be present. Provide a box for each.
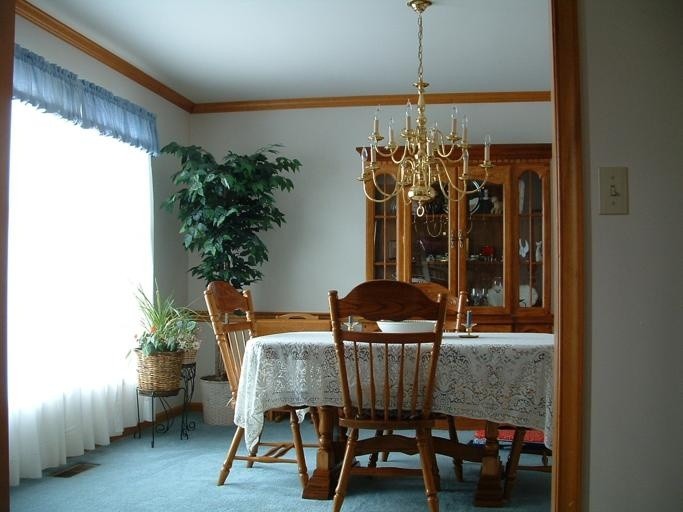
[519,285,539,308]
[486,285,503,307]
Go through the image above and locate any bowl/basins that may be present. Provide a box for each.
[376,320,437,333]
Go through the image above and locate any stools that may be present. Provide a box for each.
[133,364,198,449]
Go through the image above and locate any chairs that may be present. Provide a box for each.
[327,280,440,511]
[382,281,468,478]
[205,281,318,488]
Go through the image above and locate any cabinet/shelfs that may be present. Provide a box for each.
[355,143,554,333]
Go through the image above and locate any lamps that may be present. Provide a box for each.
[351,0,493,217]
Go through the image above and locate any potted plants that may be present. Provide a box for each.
[160,144,306,429]
[131,286,198,392]
[175,321,203,364]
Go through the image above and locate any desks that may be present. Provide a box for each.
[232,331,558,508]
